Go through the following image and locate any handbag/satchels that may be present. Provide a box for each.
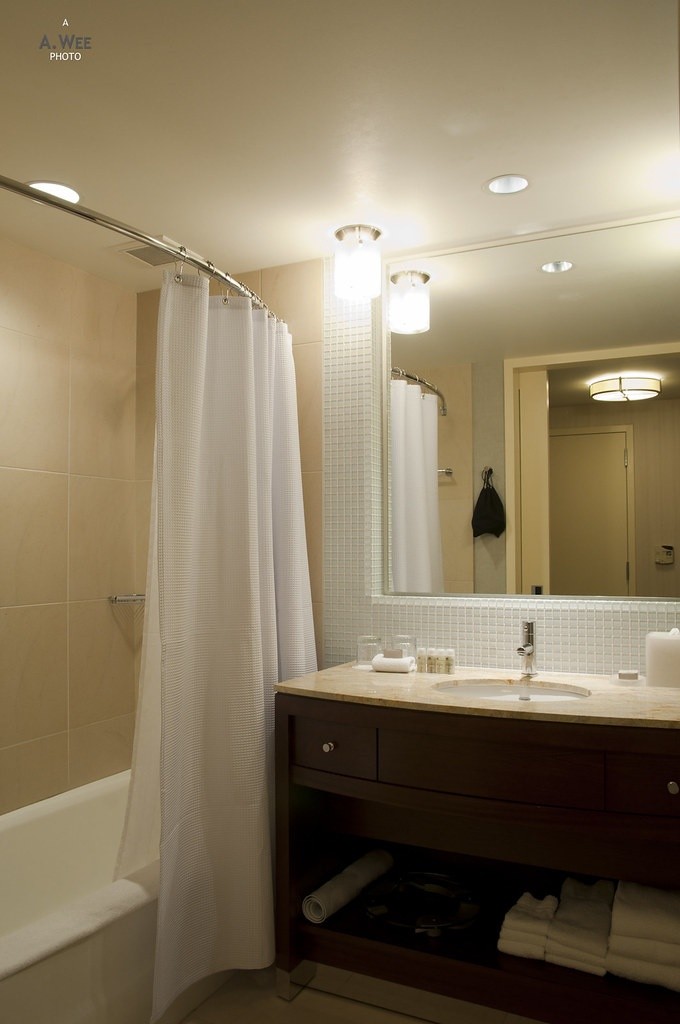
[472,469,505,538]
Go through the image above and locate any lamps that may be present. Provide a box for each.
[390,271,432,336]
[335,225,383,305]
[588,378,661,405]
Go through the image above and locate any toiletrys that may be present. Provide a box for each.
[416,648,455,674]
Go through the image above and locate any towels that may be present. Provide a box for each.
[544,877,613,977]
[372,653,417,673]
[497,892,558,960]
[605,879,680,995]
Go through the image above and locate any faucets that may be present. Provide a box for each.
[516,620,540,677]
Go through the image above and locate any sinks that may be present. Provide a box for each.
[429,678,592,703]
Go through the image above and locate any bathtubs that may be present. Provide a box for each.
[0,767,235,1024]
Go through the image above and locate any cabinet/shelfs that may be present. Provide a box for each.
[275,692,680,1024]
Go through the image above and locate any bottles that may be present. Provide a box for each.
[445,648,456,674]
[436,649,445,674]
[427,648,437,673]
[417,647,426,673]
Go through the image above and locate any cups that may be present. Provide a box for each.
[356,636,382,665]
[395,635,417,659]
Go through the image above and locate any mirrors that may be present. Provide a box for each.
[379,210,680,602]
